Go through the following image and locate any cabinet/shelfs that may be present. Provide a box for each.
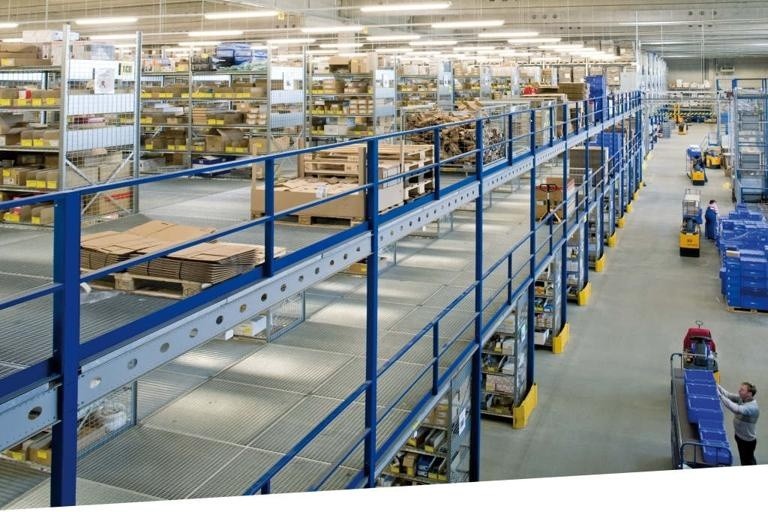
[374,145,643,488]
[0,23,521,231]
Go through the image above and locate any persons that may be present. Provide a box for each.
[704,199,720,241]
[715,381,759,466]
